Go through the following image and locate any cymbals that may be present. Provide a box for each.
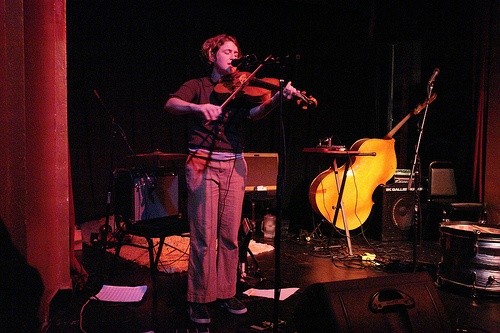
[129,152,190,161]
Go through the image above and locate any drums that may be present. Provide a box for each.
[433,220,500,302]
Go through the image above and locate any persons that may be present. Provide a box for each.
[165,34,300,324]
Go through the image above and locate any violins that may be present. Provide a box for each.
[213,71,318,110]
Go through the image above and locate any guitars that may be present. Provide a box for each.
[307,93,439,232]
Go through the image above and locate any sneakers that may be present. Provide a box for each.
[224,296,247,314]
[187,302,211,323]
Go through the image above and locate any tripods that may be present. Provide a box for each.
[83,89,152,258]
[404,85,435,265]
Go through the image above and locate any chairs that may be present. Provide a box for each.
[421,160,483,245]
[107,168,192,332]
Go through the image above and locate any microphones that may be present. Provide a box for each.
[231,54,257,68]
[427,68,440,85]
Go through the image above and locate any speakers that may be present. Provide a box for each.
[366,184,424,241]
[294,272,449,333]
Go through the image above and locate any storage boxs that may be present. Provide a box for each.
[242,153,280,191]
